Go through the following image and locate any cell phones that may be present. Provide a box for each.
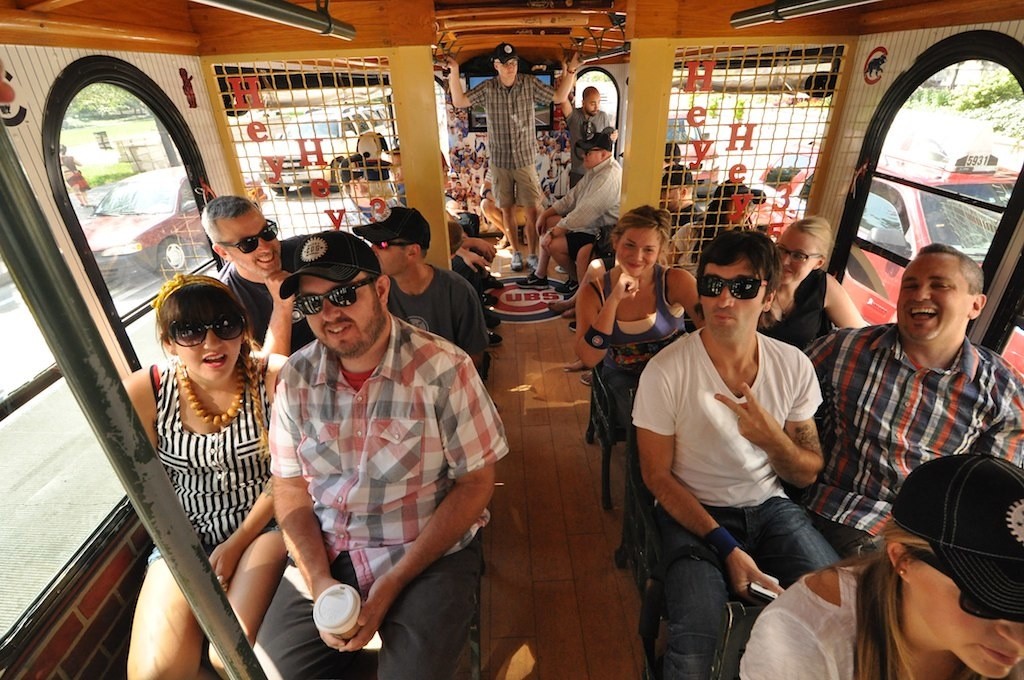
[748,574,779,601]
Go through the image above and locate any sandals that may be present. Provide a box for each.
[580,371,593,386]
[564,360,589,372]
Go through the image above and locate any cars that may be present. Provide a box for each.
[753,150,1024,376]
[665,113,721,196]
[83,164,213,283]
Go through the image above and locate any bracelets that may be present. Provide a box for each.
[706,527,739,557]
[567,69,576,74]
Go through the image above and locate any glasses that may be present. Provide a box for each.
[696,274,769,299]
[584,148,603,157]
[217,219,278,255]
[774,242,824,263]
[495,59,518,68]
[293,274,378,316]
[373,238,414,251]
[903,544,1003,620]
[166,312,245,348]
[741,198,756,213]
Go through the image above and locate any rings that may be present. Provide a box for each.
[217,575,224,584]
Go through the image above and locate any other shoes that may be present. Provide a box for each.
[546,299,578,332]
[494,240,509,249]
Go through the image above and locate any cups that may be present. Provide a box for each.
[313,584,364,640]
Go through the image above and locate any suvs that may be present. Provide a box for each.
[258,104,400,194]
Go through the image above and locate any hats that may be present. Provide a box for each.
[352,206,431,251]
[581,133,612,153]
[661,166,694,189]
[890,452,1024,623]
[715,180,767,204]
[493,43,520,64]
[358,132,382,159]
[280,232,382,300]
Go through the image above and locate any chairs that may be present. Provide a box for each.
[582,362,775,680]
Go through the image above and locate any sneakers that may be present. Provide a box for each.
[480,273,504,346]
[511,253,579,294]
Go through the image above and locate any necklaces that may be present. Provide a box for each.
[776,295,794,320]
[176,363,245,426]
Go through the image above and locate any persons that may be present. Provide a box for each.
[202,195,316,356]
[480,168,542,250]
[739,453,1024,680]
[758,217,866,351]
[338,134,504,348]
[349,207,491,371]
[660,144,767,274]
[802,244,1024,556]
[442,42,585,273]
[126,278,290,680]
[629,232,842,680]
[556,55,618,187]
[515,133,621,293]
[576,206,704,430]
[250,231,509,680]
[446,108,572,230]
